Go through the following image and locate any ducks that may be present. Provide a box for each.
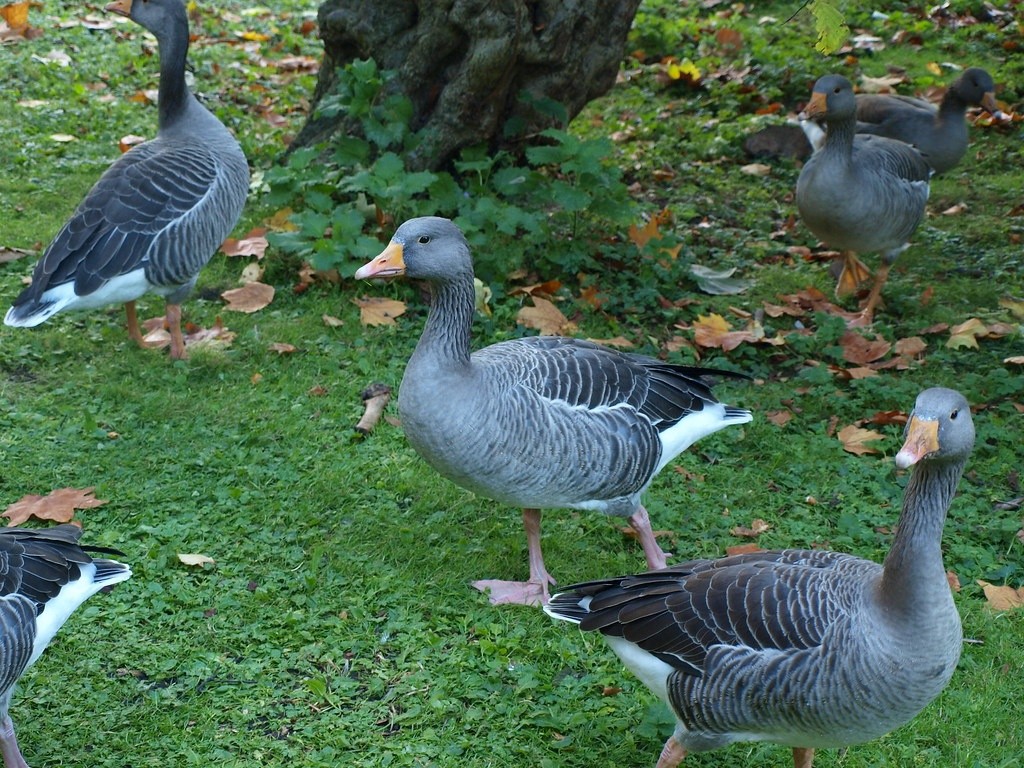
[788,66,1005,176]
[349,216,756,609]
[0,526,132,768]
[545,385,974,768]
[795,74,934,332]
[4,1,251,360]
